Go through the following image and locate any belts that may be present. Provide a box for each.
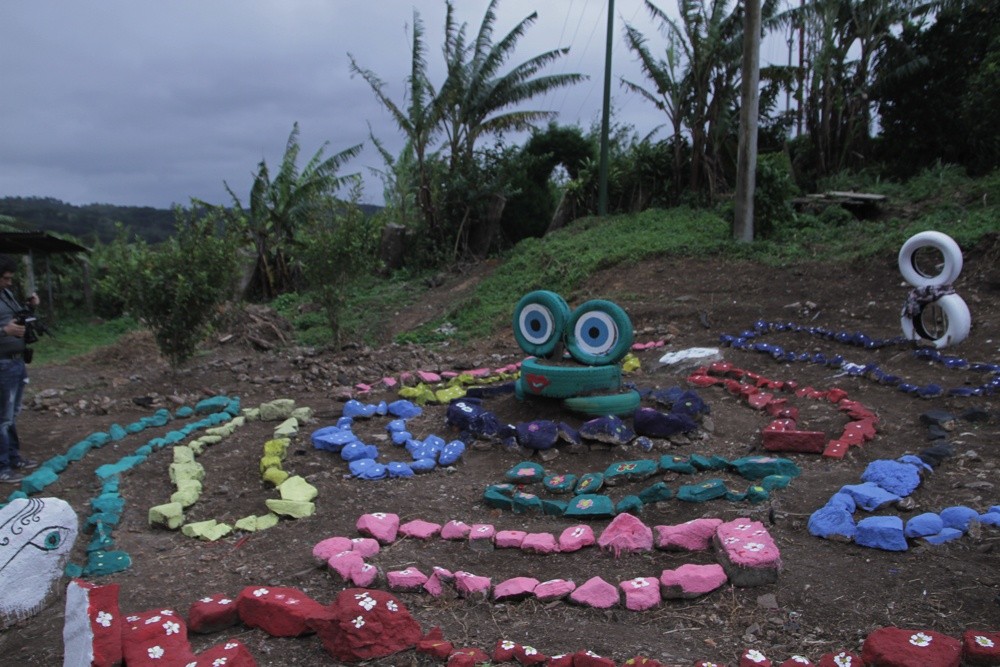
[0,350,25,361]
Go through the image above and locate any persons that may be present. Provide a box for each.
[0,254,37,483]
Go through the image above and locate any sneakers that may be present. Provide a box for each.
[0,467,25,483]
[17,459,39,469]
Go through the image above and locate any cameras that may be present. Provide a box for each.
[13,310,40,345]
